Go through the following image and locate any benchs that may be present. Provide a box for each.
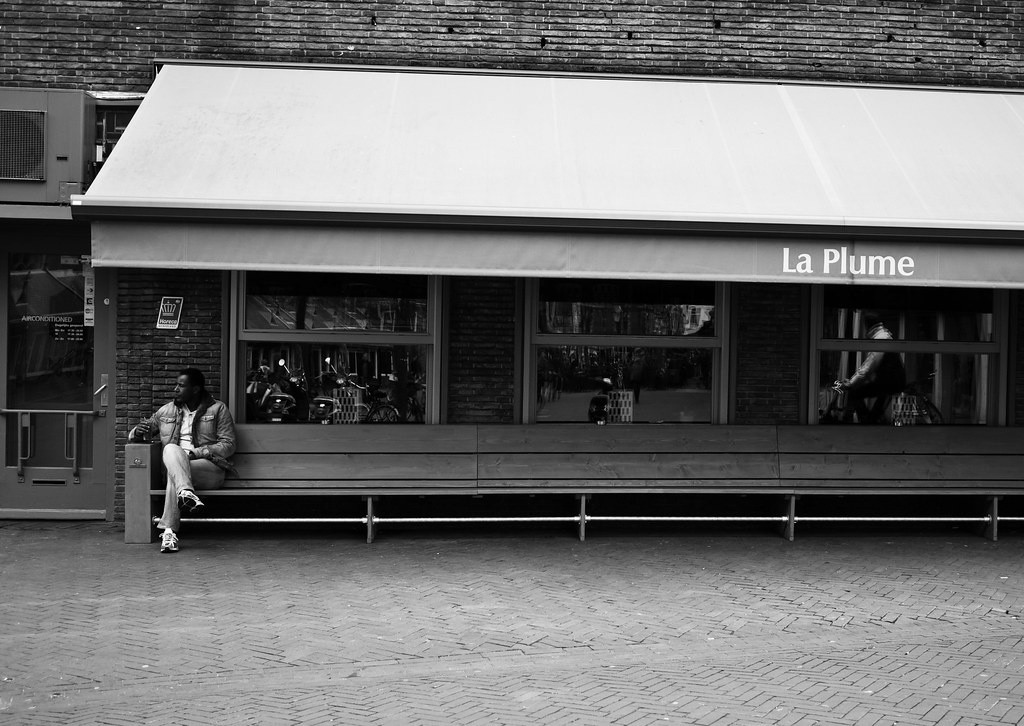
[123,423,1024,541]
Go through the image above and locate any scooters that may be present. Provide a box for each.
[246,357,350,424]
[588,376,613,424]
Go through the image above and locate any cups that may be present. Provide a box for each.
[141,420,153,442]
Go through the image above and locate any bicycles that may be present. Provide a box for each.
[819,379,945,425]
[350,380,423,423]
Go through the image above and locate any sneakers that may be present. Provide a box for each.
[177,490,205,515]
[159,532,179,552]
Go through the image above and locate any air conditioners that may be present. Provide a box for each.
[0,86,97,204]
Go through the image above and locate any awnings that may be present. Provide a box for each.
[70,59,1024,291]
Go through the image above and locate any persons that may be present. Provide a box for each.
[835,312,905,425]
[127,368,237,553]
